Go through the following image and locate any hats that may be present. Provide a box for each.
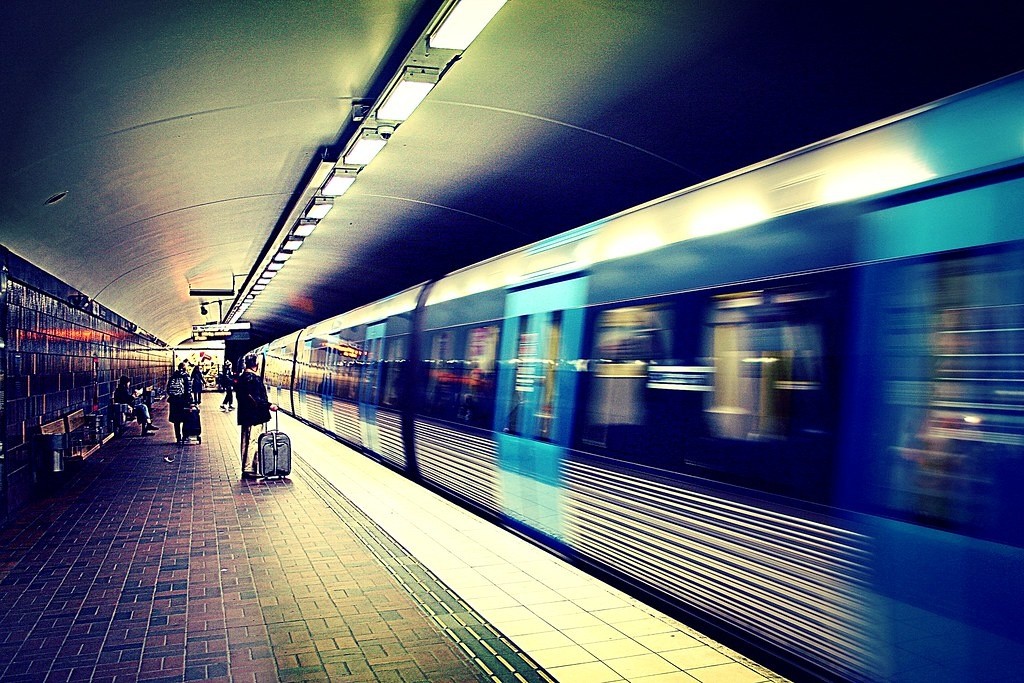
[245,354,259,367]
[225,360,232,367]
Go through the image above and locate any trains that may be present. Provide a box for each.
[235,68,1024,683]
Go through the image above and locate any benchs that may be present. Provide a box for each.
[40,385,166,463]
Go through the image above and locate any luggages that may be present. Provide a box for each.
[258,406,291,481]
[181,403,202,445]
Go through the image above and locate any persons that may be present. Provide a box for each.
[114,375,159,436]
[166,360,236,443]
[236,353,278,479]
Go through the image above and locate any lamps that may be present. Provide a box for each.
[224,0,508,322]
[189,275,235,296]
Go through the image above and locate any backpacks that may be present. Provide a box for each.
[168,374,185,397]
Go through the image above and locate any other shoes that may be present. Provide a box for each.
[218,405,226,411]
[228,406,236,411]
[241,472,262,481]
[145,423,159,430]
[141,431,155,437]
